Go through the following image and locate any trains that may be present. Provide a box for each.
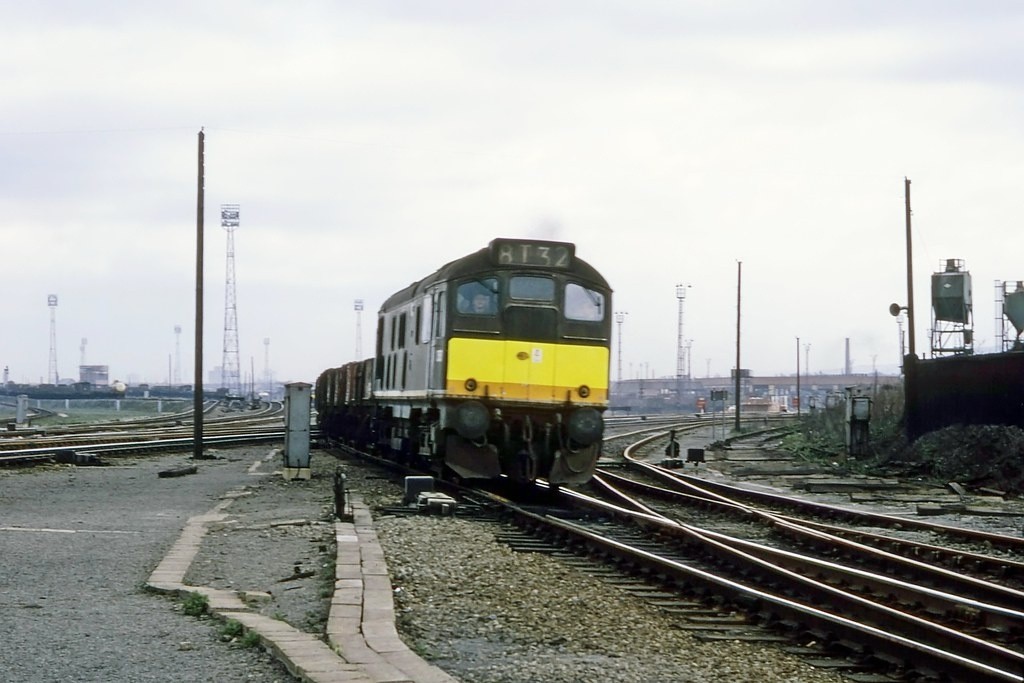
[315,238,615,485]
